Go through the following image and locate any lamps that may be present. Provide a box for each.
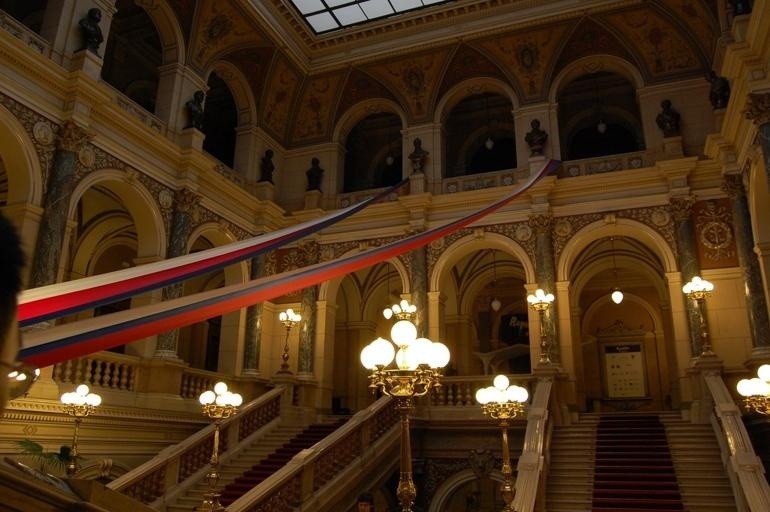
[383,261,393,319]
[490,249,501,312]
[607,237,624,305]
[392,300,417,321]
[277,308,302,375]
[736,365,770,416]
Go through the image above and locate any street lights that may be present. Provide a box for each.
[274,306,304,373]
[735,357,770,418]
[383,297,419,329]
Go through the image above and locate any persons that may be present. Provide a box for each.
[355,491,375,512]
[182,88,207,129]
[653,97,684,139]
[521,119,549,158]
[304,156,325,186]
[76,6,106,52]
[1,213,34,424]
[407,137,430,174]
[260,148,277,179]
